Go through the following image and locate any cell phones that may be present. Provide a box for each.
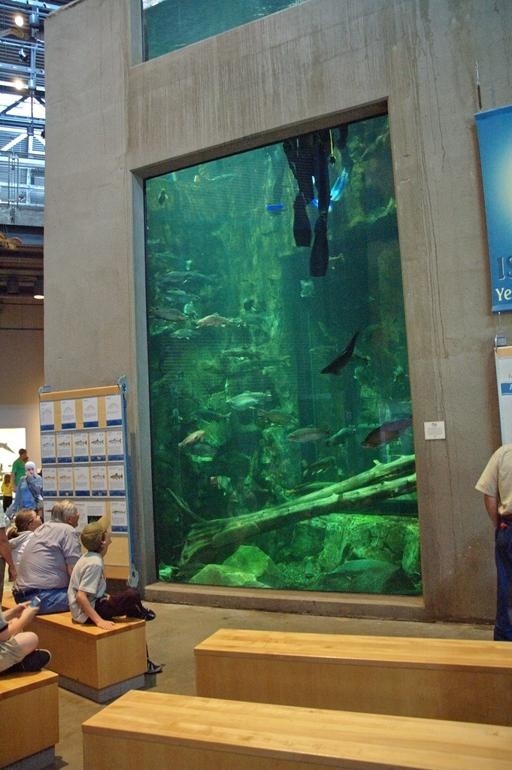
[28,596,41,610]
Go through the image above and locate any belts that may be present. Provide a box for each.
[14,588,37,600]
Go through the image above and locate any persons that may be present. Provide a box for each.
[66,514,164,674]
[0,598,53,676]
[282,123,348,276]
[0,447,44,609]
[13,500,84,617]
[474,443,512,643]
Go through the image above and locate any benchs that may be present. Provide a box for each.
[194,627,512,727]
[81,688,512,770]
[1,590,148,703]
[0,665,60,766]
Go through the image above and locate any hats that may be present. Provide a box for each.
[82,516,111,550]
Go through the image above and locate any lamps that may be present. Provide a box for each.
[17,8,39,137]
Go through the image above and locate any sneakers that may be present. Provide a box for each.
[14,649,52,673]
[137,609,155,620]
[147,660,161,673]
